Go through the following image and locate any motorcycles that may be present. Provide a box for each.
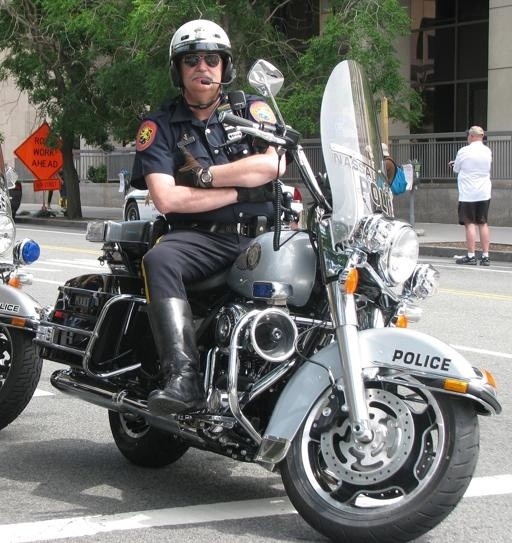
[45,60,501,542]
[0,136,53,431]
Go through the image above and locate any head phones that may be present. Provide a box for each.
[167,58,236,85]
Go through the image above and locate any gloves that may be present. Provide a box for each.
[174,147,202,186]
[235,181,285,206]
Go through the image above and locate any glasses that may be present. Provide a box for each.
[178,53,225,67]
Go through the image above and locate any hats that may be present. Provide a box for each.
[381,143,389,157]
[465,126,484,137]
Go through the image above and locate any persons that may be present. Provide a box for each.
[448,124,493,266]
[380,143,406,216]
[48,172,65,208]
[129,16,300,413]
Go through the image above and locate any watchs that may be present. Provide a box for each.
[198,166,215,189]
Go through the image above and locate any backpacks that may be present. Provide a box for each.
[384,157,407,195]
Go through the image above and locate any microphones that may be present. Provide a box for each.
[201,78,235,86]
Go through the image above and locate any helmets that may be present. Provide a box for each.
[169,19,233,66]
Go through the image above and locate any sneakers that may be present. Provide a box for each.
[480,257,490,266]
[456,256,477,265]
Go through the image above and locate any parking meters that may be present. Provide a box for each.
[405,158,421,227]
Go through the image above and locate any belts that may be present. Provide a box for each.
[170,222,271,238]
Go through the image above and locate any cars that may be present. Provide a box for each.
[122,178,302,222]
[7,178,22,217]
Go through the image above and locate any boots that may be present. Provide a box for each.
[147,297,208,415]
